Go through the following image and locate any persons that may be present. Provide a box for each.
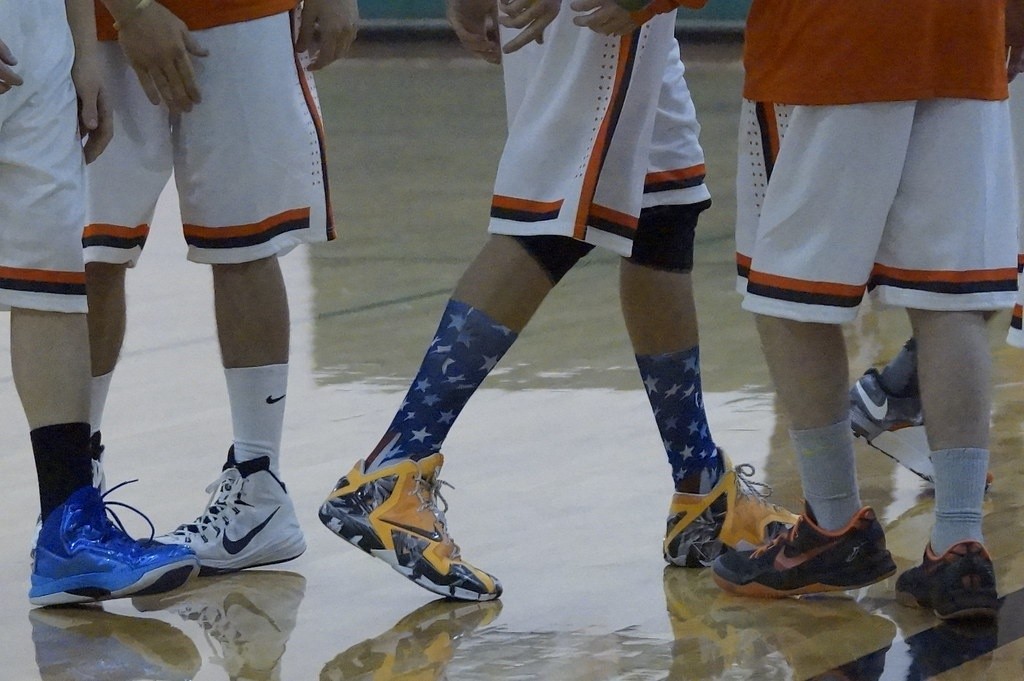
[839,1,1024,490]
[0,0,201,604]
[317,0,801,601]
[85,0,359,577]
[0,568,997,681]
[711,0,1021,618]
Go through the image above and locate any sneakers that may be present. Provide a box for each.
[661,566,743,678]
[711,506,897,601]
[25,608,201,680]
[152,446,306,572]
[317,595,503,679]
[25,479,201,608]
[848,366,994,500]
[725,592,899,681]
[896,540,1002,621]
[131,570,308,681]
[662,445,801,568]
[316,455,505,602]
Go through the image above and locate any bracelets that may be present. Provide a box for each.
[113,0,153,31]
